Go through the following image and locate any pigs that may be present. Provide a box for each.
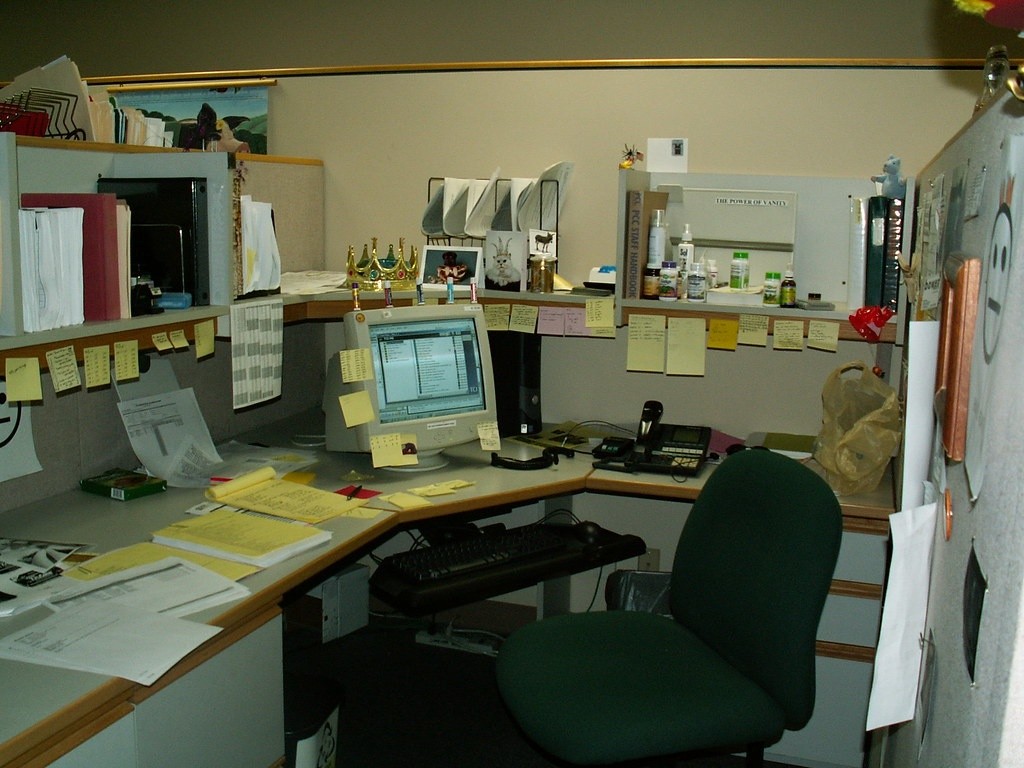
[177,102,251,152]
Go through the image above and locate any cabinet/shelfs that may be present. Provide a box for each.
[0,132,896,380]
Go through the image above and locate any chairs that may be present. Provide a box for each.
[493,447,843,768]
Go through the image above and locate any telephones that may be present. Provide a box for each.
[625,401,712,478]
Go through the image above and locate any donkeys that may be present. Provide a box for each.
[534,232,555,252]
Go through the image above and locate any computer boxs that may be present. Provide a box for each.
[488,329,542,435]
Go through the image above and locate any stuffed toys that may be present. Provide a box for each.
[871,154,906,199]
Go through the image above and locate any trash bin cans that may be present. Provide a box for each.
[614,570,679,619]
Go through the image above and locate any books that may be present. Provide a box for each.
[204,466,368,522]
[848,196,905,313]
[626,190,670,300]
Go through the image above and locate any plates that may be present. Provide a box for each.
[582,280,615,293]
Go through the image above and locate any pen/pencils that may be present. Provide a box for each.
[346,484,364,502]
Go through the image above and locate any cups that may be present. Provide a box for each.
[530,254,557,293]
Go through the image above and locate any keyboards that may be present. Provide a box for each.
[385,525,562,586]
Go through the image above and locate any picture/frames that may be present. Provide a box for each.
[937,253,983,459]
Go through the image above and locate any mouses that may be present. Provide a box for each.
[573,521,604,544]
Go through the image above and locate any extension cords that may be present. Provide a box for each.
[416,630,499,655]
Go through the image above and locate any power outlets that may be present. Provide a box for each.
[638,546,659,572]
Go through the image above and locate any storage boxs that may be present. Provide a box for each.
[287,559,370,644]
[79,465,169,499]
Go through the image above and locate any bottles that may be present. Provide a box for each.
[807,292,822,301]
[763,271,780,307]
[706,260,718,288]
[647,260,683,302]
[781,271,797,307]
[728,252,751,289]
[689,262,706,302]
[677,223,695,298]
[648,209,673,264]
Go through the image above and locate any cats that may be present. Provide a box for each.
[486,255,521,286]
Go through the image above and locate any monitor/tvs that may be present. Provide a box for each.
[326,304,496,471]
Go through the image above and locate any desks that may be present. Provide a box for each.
[1,405,894,767]
